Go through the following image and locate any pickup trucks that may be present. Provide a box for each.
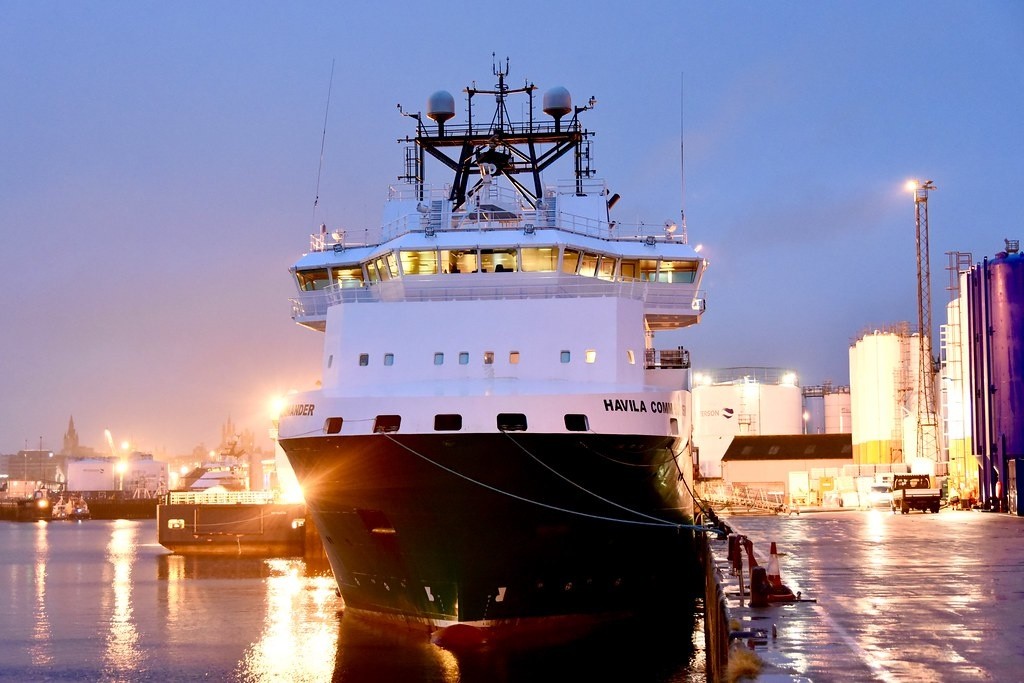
[890,474,943,514]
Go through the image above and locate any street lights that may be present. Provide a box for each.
[117,461,126,490]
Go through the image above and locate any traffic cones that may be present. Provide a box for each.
[765,542,786,594]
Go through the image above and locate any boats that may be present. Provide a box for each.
[52,496,90,519]
[277,51,709,622]
